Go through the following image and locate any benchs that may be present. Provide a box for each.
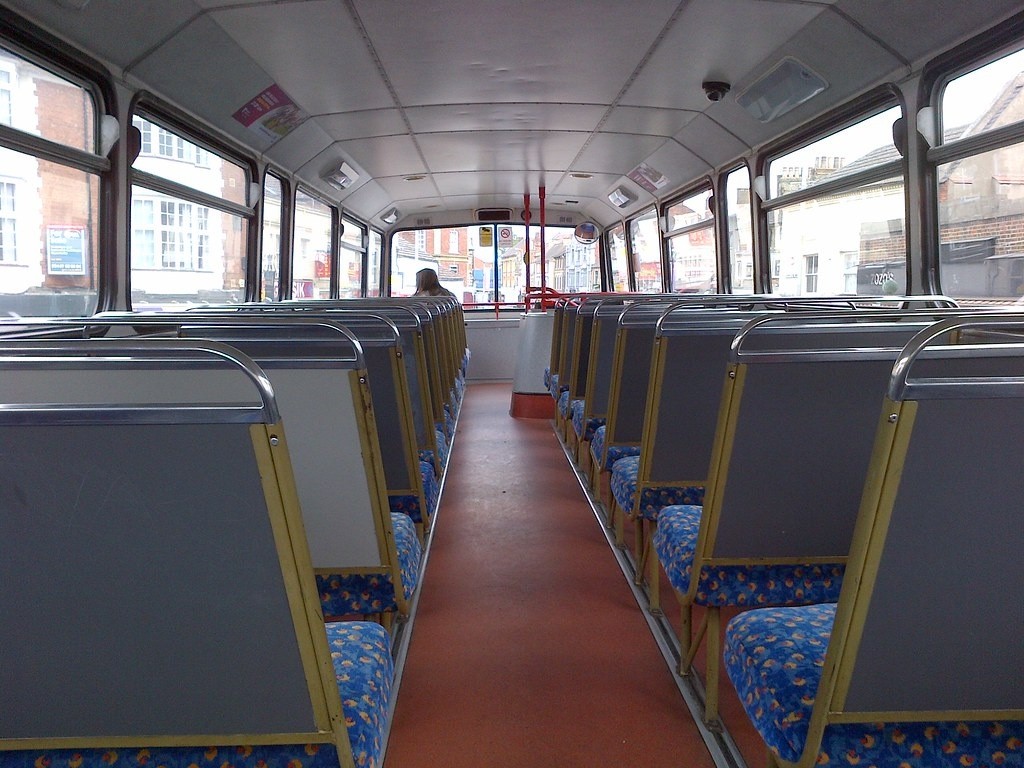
[544,291,1023,768]
[0,297,470,768]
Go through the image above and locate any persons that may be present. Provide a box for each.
[413,268,457,297]
